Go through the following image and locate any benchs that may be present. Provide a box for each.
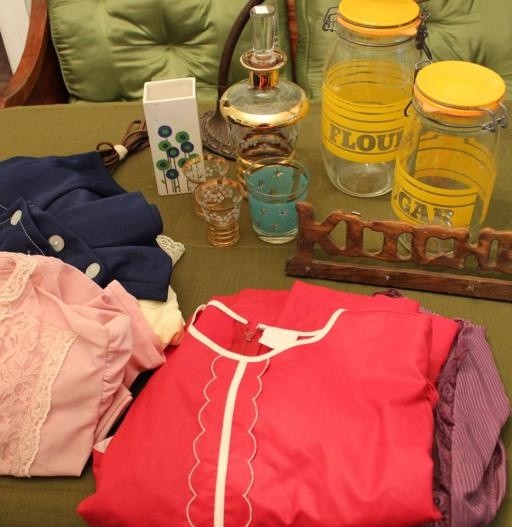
[1,0,511,105]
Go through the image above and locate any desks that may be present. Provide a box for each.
[2,103,511,527]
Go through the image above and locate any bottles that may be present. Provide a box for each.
[321,0,431,199]
[389,60,507,256]
[219,4,308,199]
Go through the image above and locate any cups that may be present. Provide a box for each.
[194,178,243,246]
[183,153,229,217]
[243,156,310,244]
